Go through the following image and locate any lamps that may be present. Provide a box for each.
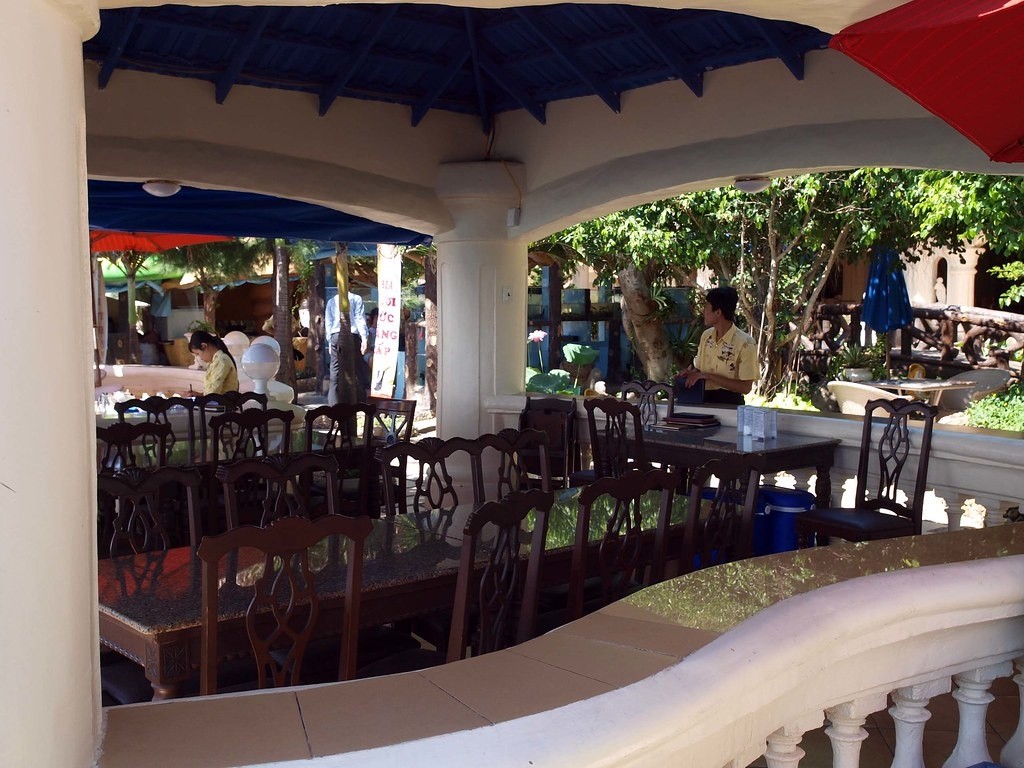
[223,330,282,395]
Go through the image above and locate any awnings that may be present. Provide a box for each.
[99,256,185,282]
[179,255,298,292]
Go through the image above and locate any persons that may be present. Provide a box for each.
[182,330,239,398]
[324,276,378,408]
[671,287,757,405]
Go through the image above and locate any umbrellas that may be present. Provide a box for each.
[860,236,914,381]
[90,229,236,365]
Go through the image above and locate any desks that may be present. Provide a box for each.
[383,484,744,650]
[596,425,842,560]
[98,435,371,562]
[858,377,978,423]
[98,521,507,707]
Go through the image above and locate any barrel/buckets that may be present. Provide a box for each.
[753,485,816,557]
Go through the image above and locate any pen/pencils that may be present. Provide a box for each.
[189,384,192,392]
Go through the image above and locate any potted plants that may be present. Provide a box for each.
[182,319,219,371]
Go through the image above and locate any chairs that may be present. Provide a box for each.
[198,514,375,697]
[680,459,739,576]
[582,396,652,484]
[826,380,916,420]
[941,368,1011,412]
[96,379,671,657]
[570,468,677,622]
[797,397,939,552]
[445,490,552,662]
